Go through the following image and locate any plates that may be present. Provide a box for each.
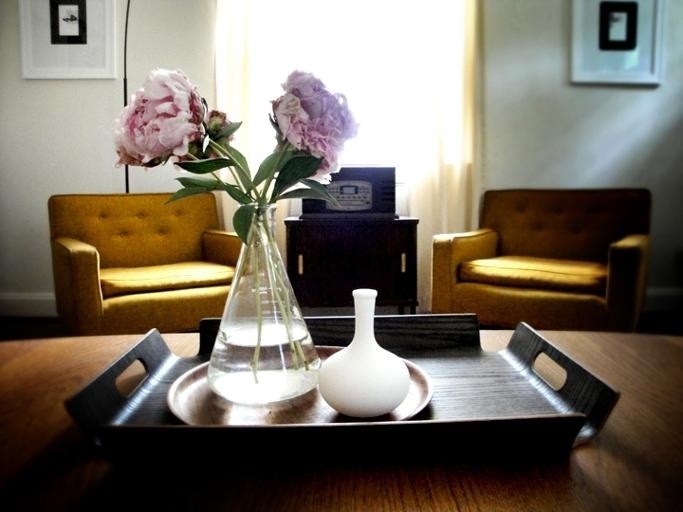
[166,343,435,426]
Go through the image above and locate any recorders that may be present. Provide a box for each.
[301,167,396,214]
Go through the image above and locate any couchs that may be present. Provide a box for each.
[48,192,242,336]
[431,188,653,330]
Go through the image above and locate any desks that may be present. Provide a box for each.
[284,214,420,315]
[0,329,682,511]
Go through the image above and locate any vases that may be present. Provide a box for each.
[317,285,412,420]
[204,202,323,410]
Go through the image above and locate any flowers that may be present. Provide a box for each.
[112,67,361,384]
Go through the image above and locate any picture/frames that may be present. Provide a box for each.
[569,0,663,86]
[18,0,117,80]
